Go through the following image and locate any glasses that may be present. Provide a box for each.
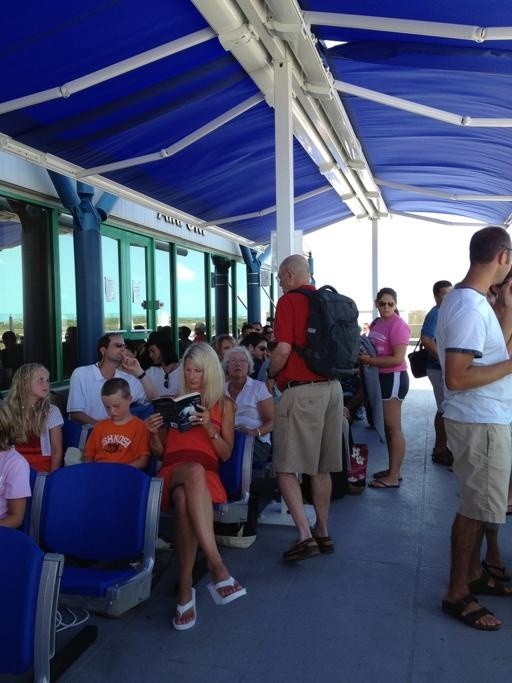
[255,345,268,351]
[164,373,169,389]
[378,301,394,307]
[112,343,128,349]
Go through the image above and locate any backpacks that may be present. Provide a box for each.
[287,285,361,378]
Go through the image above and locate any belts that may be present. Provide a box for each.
[280,379,330,393]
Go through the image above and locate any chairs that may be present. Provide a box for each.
[1,526,65,682]
[36,461,163,617]
[0,398,254,554]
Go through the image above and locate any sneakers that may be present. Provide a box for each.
[431,448,453,466]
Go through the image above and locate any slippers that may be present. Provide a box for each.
[368,478,400,489]
[172,587,197,631]
[373,471,403,482]
[206,577,248,605]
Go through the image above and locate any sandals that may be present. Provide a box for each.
[442,593,502,631]
[282,537,324,562]
[312,533,335,555]
[467,560,512,597]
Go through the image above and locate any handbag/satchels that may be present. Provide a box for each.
[408,349,427,378]
[346,444,368,487]
